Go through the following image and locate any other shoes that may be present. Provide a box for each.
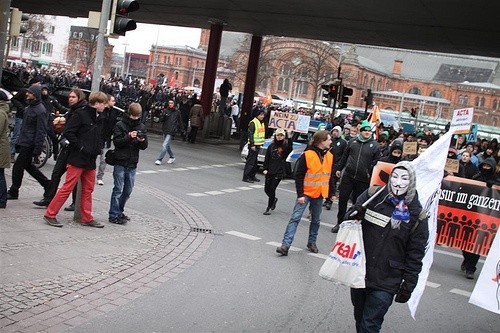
[271,198,278,210]
[97,180,104,185]
[245,177,260,183]
[263,211,271,215]
[6,193,17,199]
[331,224,340,232]
[308,242,318,253]
[34,158,40,164]
[322,199,333,209]
[276,245,289,256]
[461,261,474,279]
[155,160,161,165]
[167,158,175,164]
[33,197,132,228]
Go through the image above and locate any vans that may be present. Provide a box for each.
[343,109,399,131]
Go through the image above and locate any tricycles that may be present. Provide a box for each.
[8,111,68,168]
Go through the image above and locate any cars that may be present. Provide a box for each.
[52,86,125,133]
[241,127,326,180]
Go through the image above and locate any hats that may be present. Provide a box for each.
[275,128,285,137]
[343,121,430,155]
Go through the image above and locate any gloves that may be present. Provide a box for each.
[395,290,411,303]
[33,145,41,155]
[347,204,365,220]
[487,180,495,187]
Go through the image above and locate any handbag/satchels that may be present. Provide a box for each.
[318,220,366,289]
[105,150,118,165]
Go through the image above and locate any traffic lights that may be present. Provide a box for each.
[321,84,353,109]
[109,0,138,36]
[411,108,416,118]
[8,8,29,37]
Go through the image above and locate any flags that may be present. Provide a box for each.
[406,128,455,319]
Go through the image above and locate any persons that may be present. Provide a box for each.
[153,98,184,165]
[242,108,265,183]
[343,161,429,333]
[6,84,49,200]
[108,102,148,224]
[42,91,108,228]
[0,56,500,280]
[276,129,337,256]
[0,88,11,208]
[331,120,381,233]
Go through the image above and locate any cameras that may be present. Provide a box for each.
[137,130,143,139]
